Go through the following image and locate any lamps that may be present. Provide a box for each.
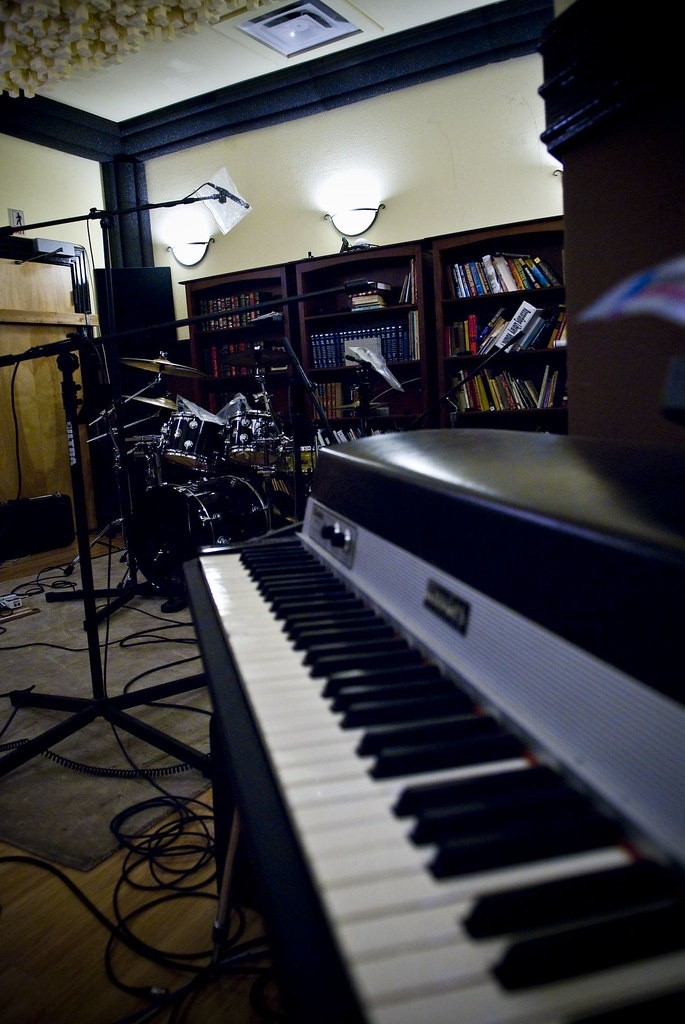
[166,238,215,267]
[324,203,386,237]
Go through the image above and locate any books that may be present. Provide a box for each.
[309,259,421,447]
[443,252,569,412]
[200,292,290,415]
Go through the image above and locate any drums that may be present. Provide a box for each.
[160,408,227,475]
[223,408,274,462]
[129,473,271,597]
[272,443,320,475]
[121,434,169,493]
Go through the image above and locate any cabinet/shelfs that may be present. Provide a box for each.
[178,214,570,529]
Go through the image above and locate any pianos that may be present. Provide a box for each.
[177,423,682,1024]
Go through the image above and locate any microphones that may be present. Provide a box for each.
[345,354,374,369]
[207,182,249,209]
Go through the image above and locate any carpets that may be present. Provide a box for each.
[0,547,212,875]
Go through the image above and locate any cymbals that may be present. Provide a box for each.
[121,394,181,410]
[116,356,205,379]
